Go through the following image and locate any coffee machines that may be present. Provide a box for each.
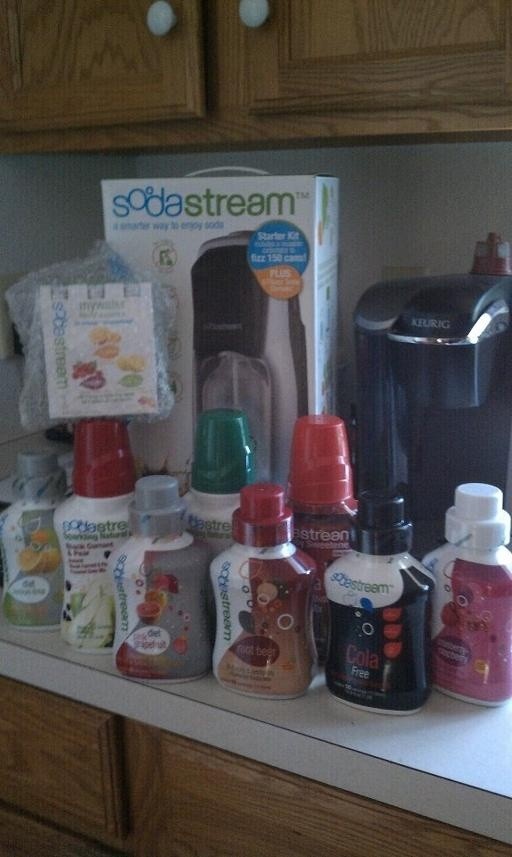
[189,222,304,489]
[350,273,509,557]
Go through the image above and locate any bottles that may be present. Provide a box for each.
[324,490,435,714]
[177,410,258,555]
[53,415,139,654]
[282,416,360,665]
[470,230,511,279]
[103,475,213,678]
[213,484,318,699]
[0,451,68,628]
[423,483,511,707]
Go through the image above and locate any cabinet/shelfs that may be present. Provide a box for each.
[2,0,512,160]
[1,673,510,857]
